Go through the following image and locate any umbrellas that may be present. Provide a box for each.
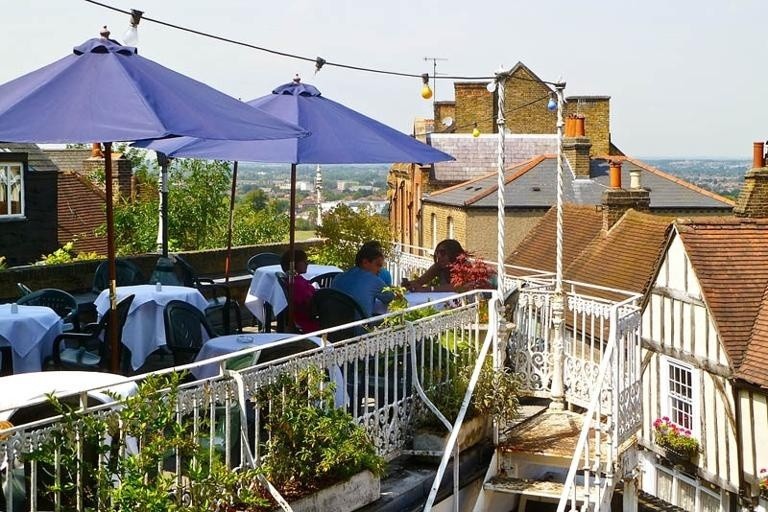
[128,75,456,326]
[0,26,311,370]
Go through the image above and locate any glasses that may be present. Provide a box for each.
[435,249,448,258]
[302,259,308,265]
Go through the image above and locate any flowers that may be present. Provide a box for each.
[759,466,768,488]
[654,414,695,437]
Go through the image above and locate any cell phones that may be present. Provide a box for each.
[402,278,407,283]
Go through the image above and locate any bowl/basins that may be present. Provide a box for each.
[236,335,253,343]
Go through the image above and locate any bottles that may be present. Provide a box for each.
[156,282,161,293]
[11,303,18,313]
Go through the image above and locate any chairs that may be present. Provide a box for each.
[16,289,82,349]
[92,248,501,389]
[43,294,136,376]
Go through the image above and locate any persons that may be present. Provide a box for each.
[362,243,392,287]
[281,250,321,333]
[331,246,403,335]
[401,239,484,308]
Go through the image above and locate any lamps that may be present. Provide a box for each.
[122,7,555,138]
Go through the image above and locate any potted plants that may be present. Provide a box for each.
[376,273,521,458]
[22,366,387,512]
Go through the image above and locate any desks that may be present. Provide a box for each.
[1,304,64,373]
[2,371,142,421]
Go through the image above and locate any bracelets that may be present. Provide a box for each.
[430,285,434,291]
